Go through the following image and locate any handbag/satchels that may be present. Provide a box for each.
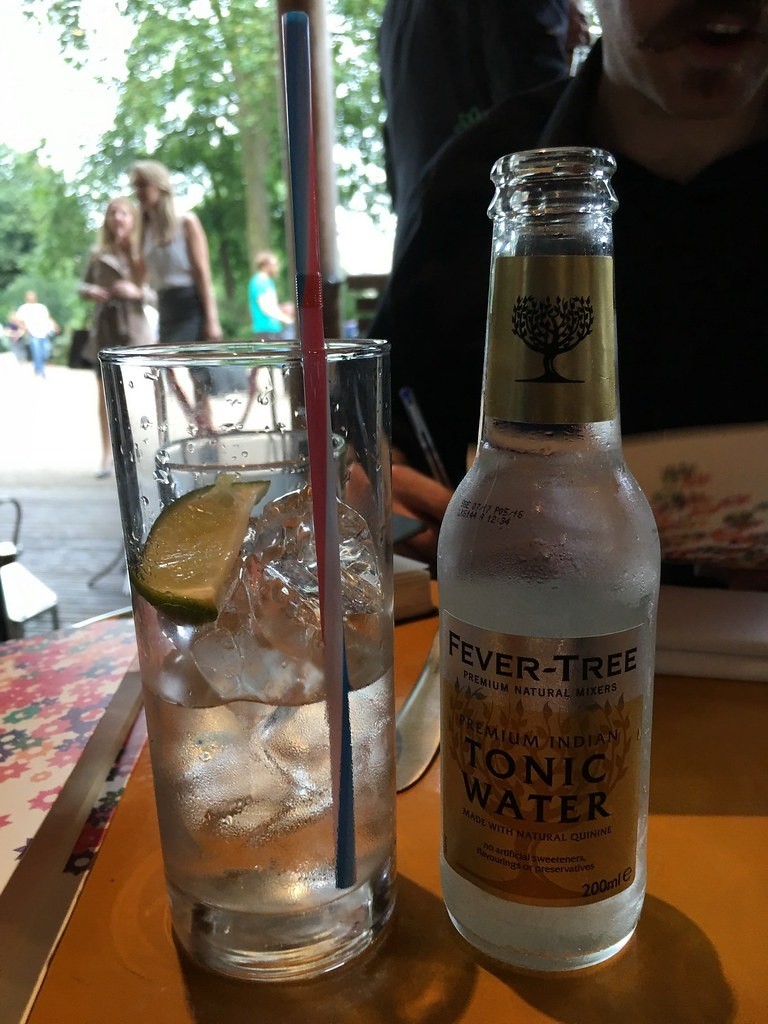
[66,286,97,369]
[206,365,248,396]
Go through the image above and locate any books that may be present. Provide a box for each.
[392,514,439,625]
[655,584,767,681]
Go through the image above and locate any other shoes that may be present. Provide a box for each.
[96,469,112,479]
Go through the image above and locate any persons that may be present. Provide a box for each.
[249,251,296,341]
[117,159,223,434]
[333,0,768,587]
[16,290,52,375]
[78,197,158,478]
[377,0,589,231]
[4,311,28,362]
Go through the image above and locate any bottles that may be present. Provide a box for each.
[436,148,662,973]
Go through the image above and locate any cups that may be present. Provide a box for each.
[98,339,396,983]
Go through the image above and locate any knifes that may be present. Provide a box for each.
[394,629,441,793]
